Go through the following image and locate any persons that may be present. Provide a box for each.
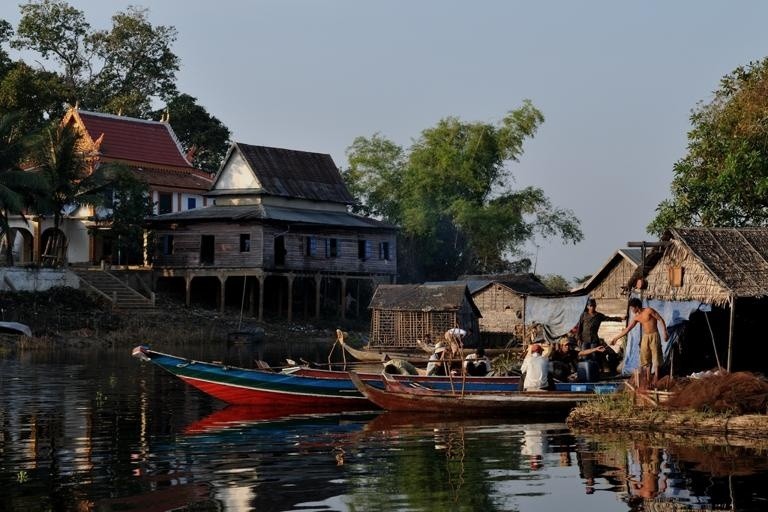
[464,347,494,377]
[444,327,473,359]
[575,299,628,362]
[520,345,550,392]
[383,359,419,376]
[550,336,607,383]
[609,297,670,389]
[425,342,450,377]
[519,429,670,512]
[424,335,434,346]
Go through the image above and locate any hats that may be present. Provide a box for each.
[434,341,450,354]
[530,344,544,352]
[587,299,596,305]
[559,337,570,345]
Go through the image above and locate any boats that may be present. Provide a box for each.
[130,328,626,417]
